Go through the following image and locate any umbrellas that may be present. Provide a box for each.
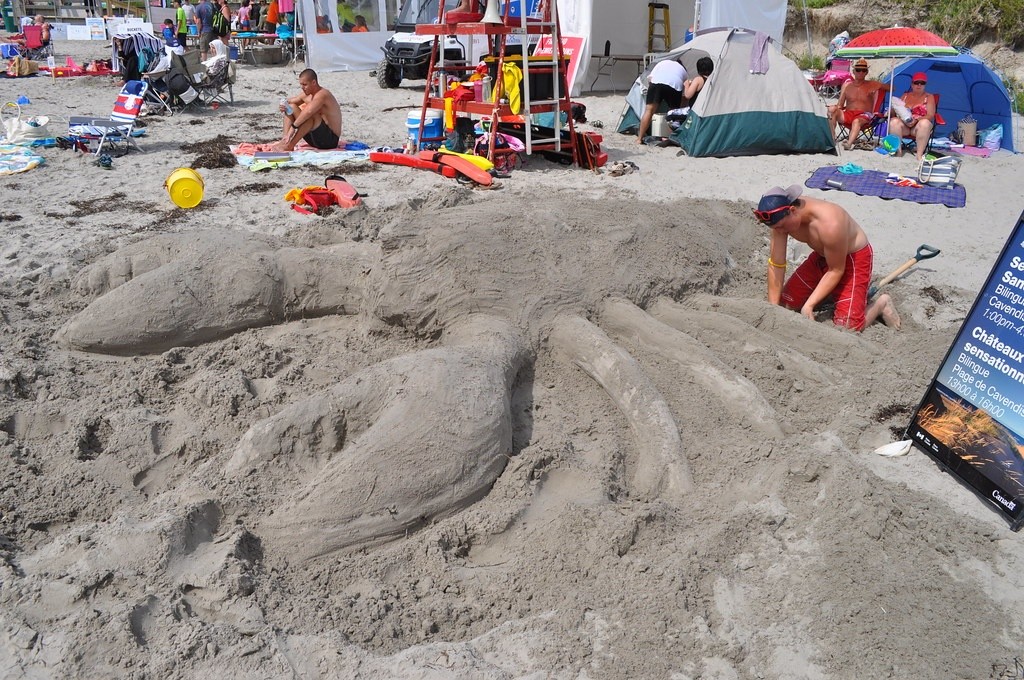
[835,23,959,137]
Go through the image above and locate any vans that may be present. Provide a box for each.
[376,1,541,89]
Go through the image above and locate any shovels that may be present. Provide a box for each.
[866,244,940,307]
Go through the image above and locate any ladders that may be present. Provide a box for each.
[648,2,671,53]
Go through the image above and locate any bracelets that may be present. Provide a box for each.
[768,257,786,268]
[291,123,298,129]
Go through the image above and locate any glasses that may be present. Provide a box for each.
[855,68,868,72]
[753,206,795,220]
[912,81,926,85]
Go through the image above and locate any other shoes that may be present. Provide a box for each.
[99,154,112,169]
[854,138,876,150]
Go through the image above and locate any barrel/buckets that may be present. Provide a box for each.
[164,167,205,208]
[405,110,442,148]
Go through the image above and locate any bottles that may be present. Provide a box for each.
[482,77,492,102]
[281,99,294,115]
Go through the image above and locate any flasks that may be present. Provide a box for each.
[827,180,843,188]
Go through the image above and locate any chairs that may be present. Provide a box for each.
[177,49,234,108]
[23,25,54,60]
[882,90,946,158]
[836,89,886,146]
[68,80,149,157]
[823,60,852,100]
[643,53,659,71]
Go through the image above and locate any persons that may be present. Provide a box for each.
[0,13,54,48]
[162,0,368,61]
[884,71,937,162]
[631,55,714,146]
[828,58,896,150]
[200,39,228,88]
[752,184,901,334]
[272,69,342,153]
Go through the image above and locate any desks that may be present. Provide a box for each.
[231,34,303,67]
[591,54,649,94]
[186,35,200,49]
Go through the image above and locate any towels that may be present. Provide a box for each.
[491,61,523,116]
[749,30,770,75]
[112,31,162,73]
[225,139,404,172]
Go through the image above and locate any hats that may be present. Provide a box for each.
[912,72,927,83]
[852,57,869,68]
[755,185,804,226]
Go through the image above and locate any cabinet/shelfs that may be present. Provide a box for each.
[418,0,584,171]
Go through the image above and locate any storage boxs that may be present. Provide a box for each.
[246,45,282,64]
[534,112,567,129]
[651,113,671,137]
[484,54,570,101]
[405,108,444,150]
[1,43,19,59]
[229,43,238,61]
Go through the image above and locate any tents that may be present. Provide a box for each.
[881,47,1016,153]
[614,25,839,154]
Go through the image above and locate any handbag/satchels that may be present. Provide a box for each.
[957,119,978,146]
[918,153,962,189]
[210,3,229,37]
[876,134,900,155]
[242,15,249,26]
[978,123,1003,152]
[0,101,50,146]
[892,96,912,124]
